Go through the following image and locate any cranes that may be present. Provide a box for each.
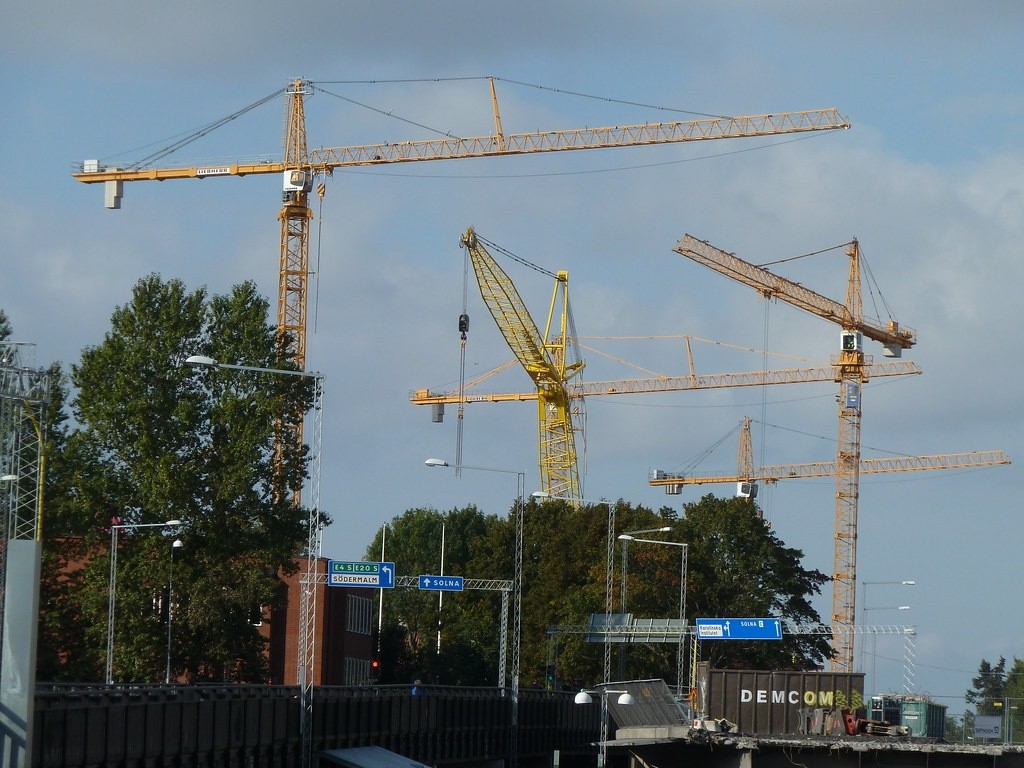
[671,233,917,672]
[409,225,925,512]
[70,75,852,528]
[649,415,1014,504]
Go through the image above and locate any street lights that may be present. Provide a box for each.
[575,688,636,768]
[106,520,182,684]
[861,606,910,675]
[186,356,325,768]
[532,490,615,690]
[424,458,526,766]
[859,580,915,675]
[872,629,916,696]
[618,527,674,681]
[166,539,184,685]
[618,534,688,699]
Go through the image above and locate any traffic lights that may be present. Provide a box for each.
[370,657,382,677]
[546,665,555,690]
